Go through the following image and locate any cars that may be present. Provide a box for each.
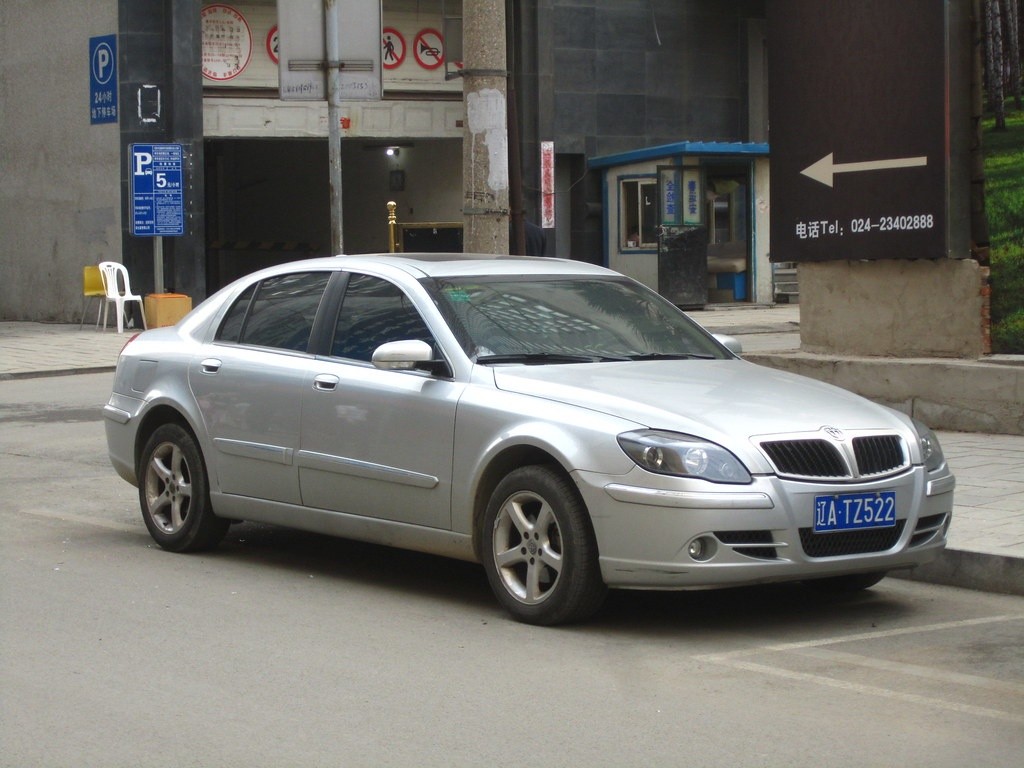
[104,251,957,629]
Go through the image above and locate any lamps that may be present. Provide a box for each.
[387,148,399,156]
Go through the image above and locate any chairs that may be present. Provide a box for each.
[79,267,131,331]
[97,262,147,334]
